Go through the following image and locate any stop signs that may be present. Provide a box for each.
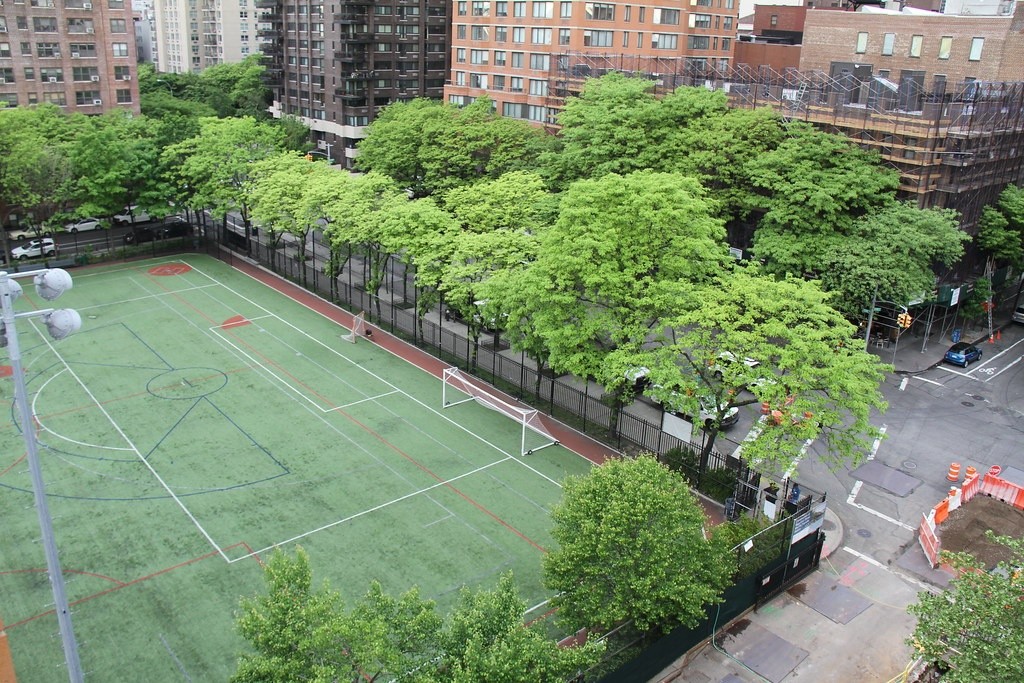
[988,464,1001,478]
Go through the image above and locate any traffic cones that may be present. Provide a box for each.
[988,333,994,343]
[996,330,1001,339]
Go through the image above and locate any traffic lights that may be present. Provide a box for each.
[896,313,912,328]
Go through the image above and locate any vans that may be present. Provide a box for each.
[113,205,166,226]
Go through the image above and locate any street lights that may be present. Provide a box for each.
[0,268,84,683]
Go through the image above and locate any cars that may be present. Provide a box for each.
[11,238,55,261]
[650,377,740,431]
[64,216,108,235]
[157,221,194,239]
[624,364,653,390]
[470,299,510,331]
[122,227,159,245]
[708,350,778,392]
[944,341,982,368]
[9,224,52,241]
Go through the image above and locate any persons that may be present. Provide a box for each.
[763,483,780,505]
[788,483,801,503]
